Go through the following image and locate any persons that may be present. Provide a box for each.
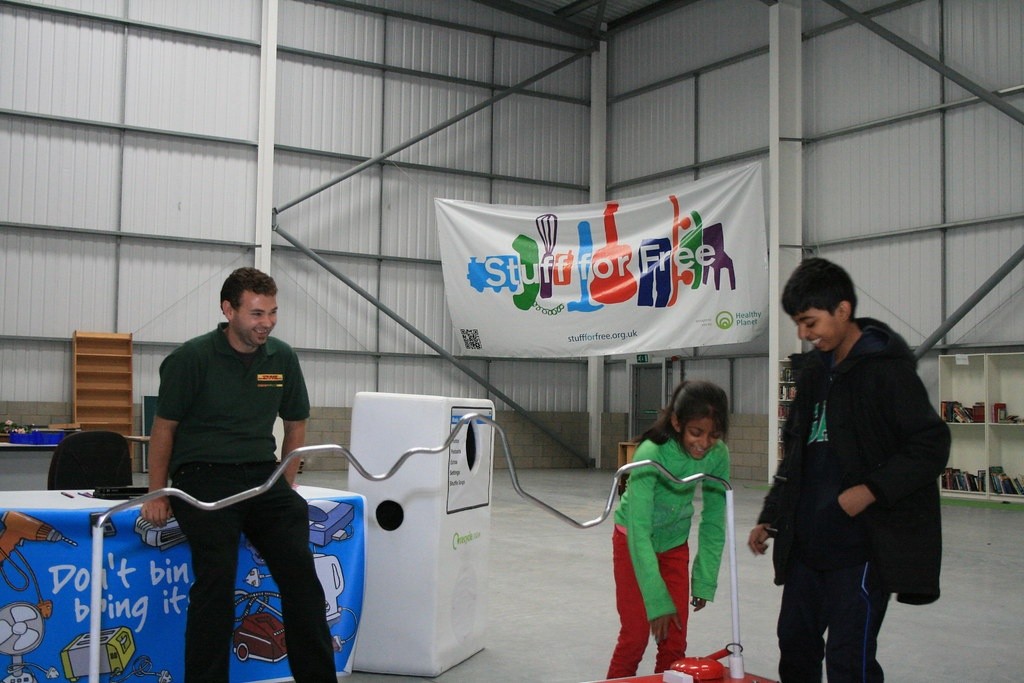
[747,258,952,683]
[140,267,338,683]
[606,380,731,678]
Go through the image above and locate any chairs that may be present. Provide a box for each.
[47,430,134,490]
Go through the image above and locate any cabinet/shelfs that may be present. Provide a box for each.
[776,359,796,462]
[937,352,1024,504]
[71,330,134,472]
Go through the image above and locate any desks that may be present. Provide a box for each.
[0,442,58,491]
[0,485,369,683]
[618,441,639,487]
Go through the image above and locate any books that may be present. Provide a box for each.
[93,487,149,500]
[778,368,798,459]
[942,466,1024,496]
[941,401,1021,423]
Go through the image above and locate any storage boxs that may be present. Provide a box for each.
[9,431,65,445]
